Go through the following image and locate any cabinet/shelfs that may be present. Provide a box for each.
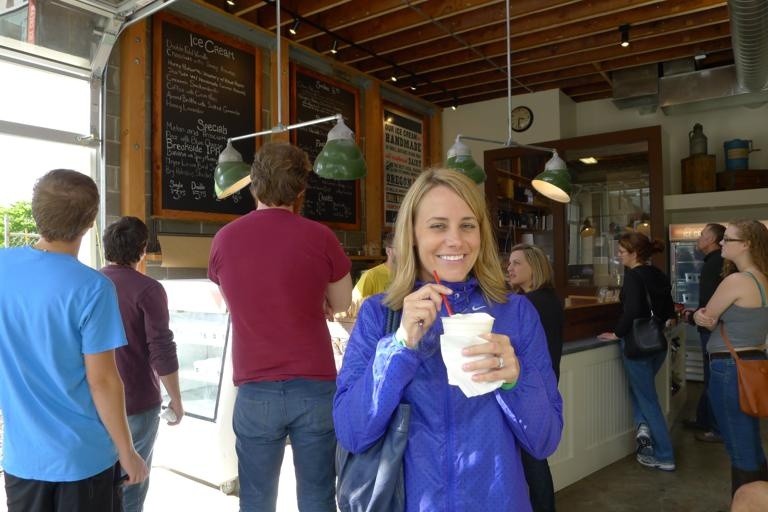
[498,164,549,253]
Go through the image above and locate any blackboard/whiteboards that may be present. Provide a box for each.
[290,63,362,230]
[151,11,263,221]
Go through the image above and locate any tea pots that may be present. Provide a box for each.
[687,121,708,156]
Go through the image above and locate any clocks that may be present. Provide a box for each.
[509,106,533,132]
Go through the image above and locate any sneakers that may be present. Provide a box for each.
[636,448,676,471]
[680,416,697,429]
[696,431,719,442]
[636,425,653,453]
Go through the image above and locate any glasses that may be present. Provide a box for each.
[721,235,746,243]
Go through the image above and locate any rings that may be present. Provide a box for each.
[498,357,504,368]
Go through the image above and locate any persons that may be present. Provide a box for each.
[0,168,152,511]
[596,231,678,473]
[337,230,397,318]
[332,167,566,512]
[729,479,768,512]
[693,216,768,495]
[207,139,354,511]
[679,222,739,445]
[506,241,565,511]
[97,215,185,510]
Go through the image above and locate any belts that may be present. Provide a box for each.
[708,351,764,360]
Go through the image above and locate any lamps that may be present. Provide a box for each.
[445,0,572,203]
[213,1,366,199]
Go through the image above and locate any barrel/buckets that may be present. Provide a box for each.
[723,140,749,171]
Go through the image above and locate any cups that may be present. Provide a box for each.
[440,313,495,385]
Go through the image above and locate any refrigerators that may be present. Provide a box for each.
[669,221,732,384]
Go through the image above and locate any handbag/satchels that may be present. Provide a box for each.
[737,359,768,417]
[335,397,411,512]
[624,317,667,358]
